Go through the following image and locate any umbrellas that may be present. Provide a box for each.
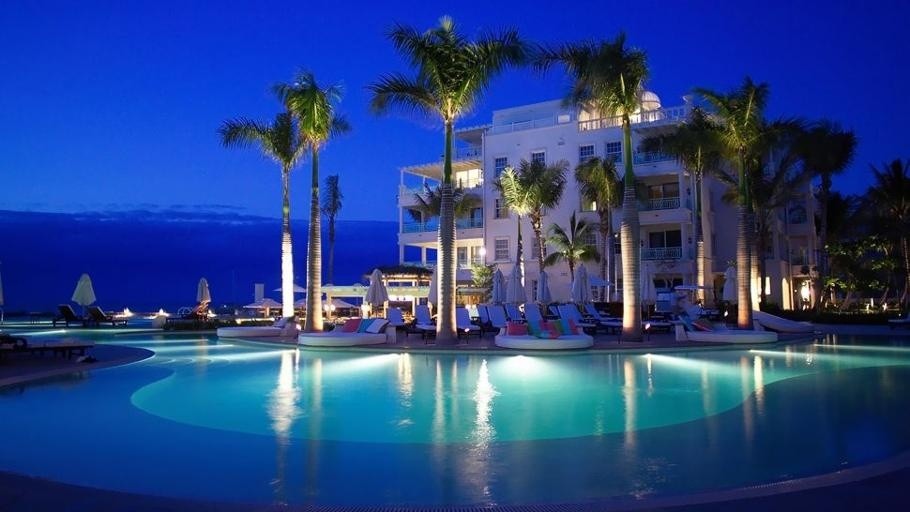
[570,262,593,305]
[674,283,713,304]
[506,264,525,306]
[428,264,437,307]
[536,270,552,315]
[70,272,96,319]
[492,268,506,305]
[640,264,657,320]
[196,276,211,319]
[365,269,389,319]
[589,272,616,287]
[722,265,738,319]
[272,282,306,293]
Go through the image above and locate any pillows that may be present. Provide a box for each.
[342,318,391,333]
[274,317,290,328]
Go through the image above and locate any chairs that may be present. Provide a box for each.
[385,300,672,346]
[52,303,129,329]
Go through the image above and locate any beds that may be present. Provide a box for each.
[675,322,779,346]
[216,321,296,338]
[298,323,389,347]
